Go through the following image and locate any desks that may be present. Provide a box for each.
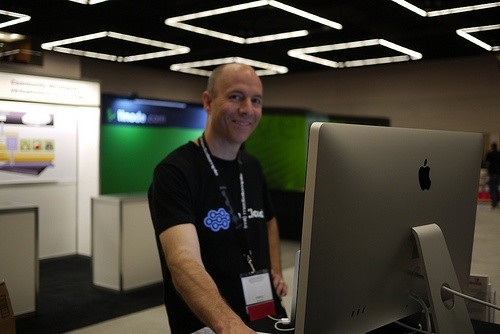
[91,197,163,292]
[0,202,40,317]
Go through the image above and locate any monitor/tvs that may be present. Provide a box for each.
[241,108,327,193]
[98,93,207,197]
[294,123,483,334]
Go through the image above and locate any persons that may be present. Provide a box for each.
[146,62,290,334]
[483,142,500,210]
